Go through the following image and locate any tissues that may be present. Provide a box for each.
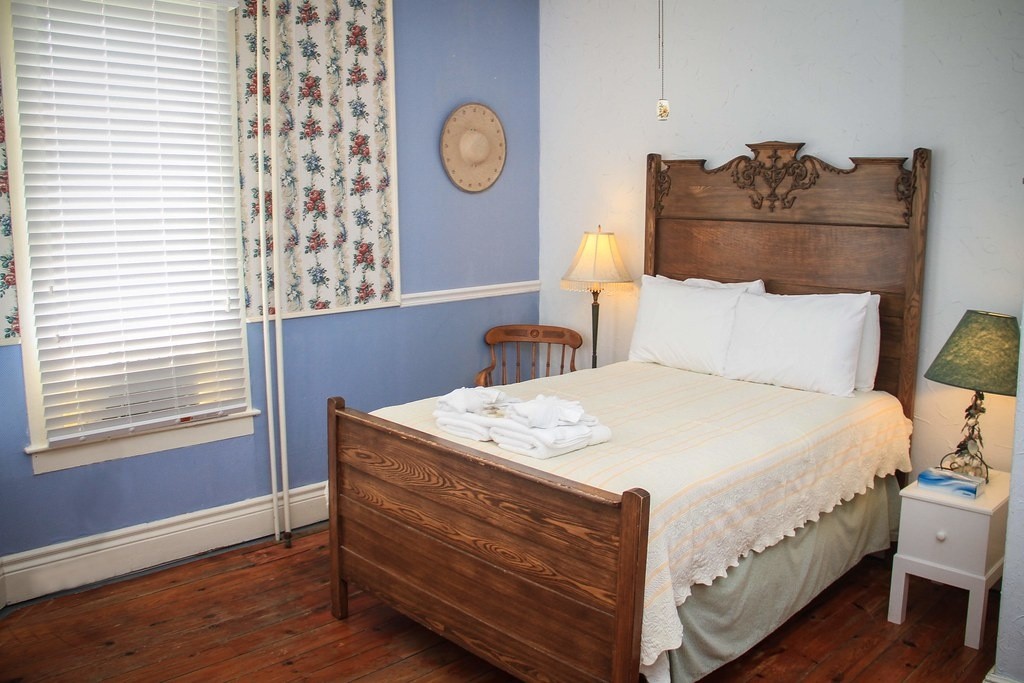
[916,467,986,500]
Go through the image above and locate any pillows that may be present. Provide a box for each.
[765,292,883,394]
[722,291,871,397]
[627,273,749,376]
[655,273,766,295]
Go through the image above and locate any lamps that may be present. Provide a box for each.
[562,225,638,368]
[927,309,1020,484]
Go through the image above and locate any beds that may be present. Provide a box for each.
[328,139,933,683]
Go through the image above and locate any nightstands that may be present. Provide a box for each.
[886,457,1011,647]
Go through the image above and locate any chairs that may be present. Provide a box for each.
[475,324,583,387]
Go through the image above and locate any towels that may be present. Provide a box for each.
[431,385,612,461]
